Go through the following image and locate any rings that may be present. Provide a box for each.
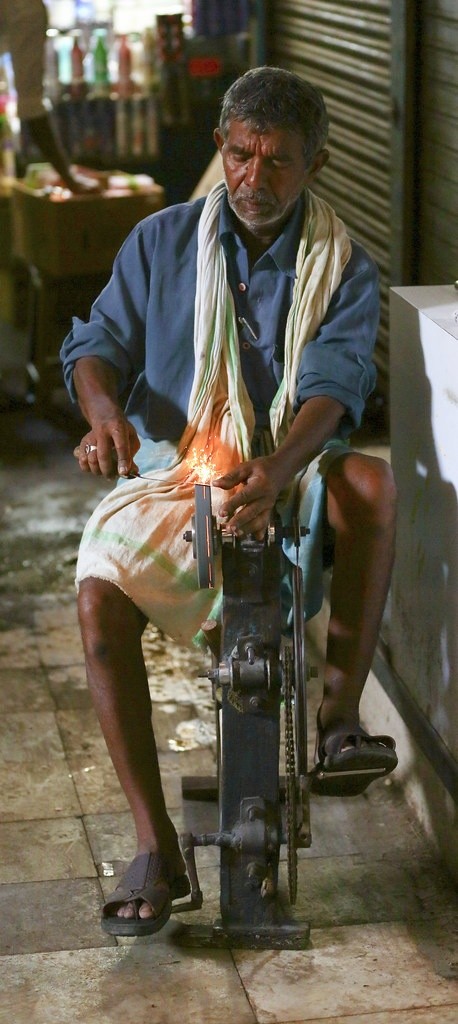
[85,445,97,453]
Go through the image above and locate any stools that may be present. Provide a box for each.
[19,264,114,417]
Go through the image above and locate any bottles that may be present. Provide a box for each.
[95,38,107,93]
[71,41,82,78]
[118,38,130,94]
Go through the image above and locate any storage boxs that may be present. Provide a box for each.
[10,176,163,270]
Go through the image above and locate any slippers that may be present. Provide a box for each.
[310,727,399,797]
[102,851,192,937]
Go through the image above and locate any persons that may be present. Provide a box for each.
[0,0,102,410]
[60,67,399,937]
